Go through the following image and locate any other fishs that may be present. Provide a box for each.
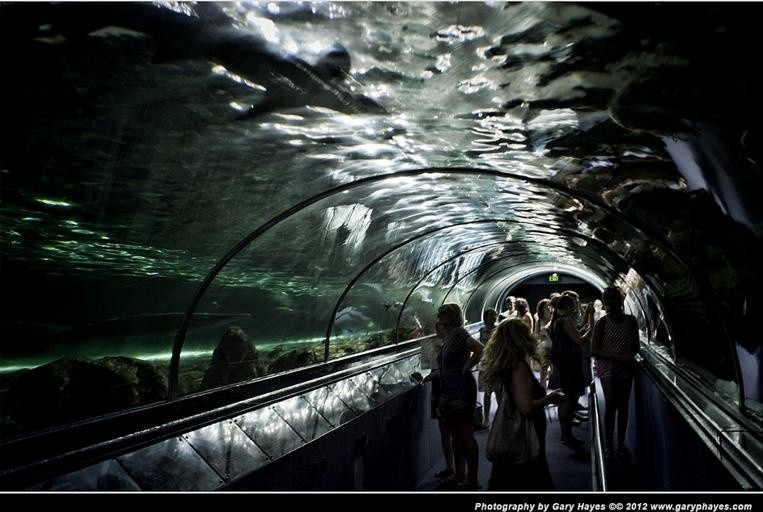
[0,2,388,134]
[0,313,252,363]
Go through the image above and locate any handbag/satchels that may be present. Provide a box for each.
[549,338,585,363]
[485,397,545,467]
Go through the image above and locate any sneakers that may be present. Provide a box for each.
[433,467,480,491]
[561,436,585,446]
[603,444,629,460]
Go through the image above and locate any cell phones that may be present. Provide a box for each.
[588,304,593,308]
